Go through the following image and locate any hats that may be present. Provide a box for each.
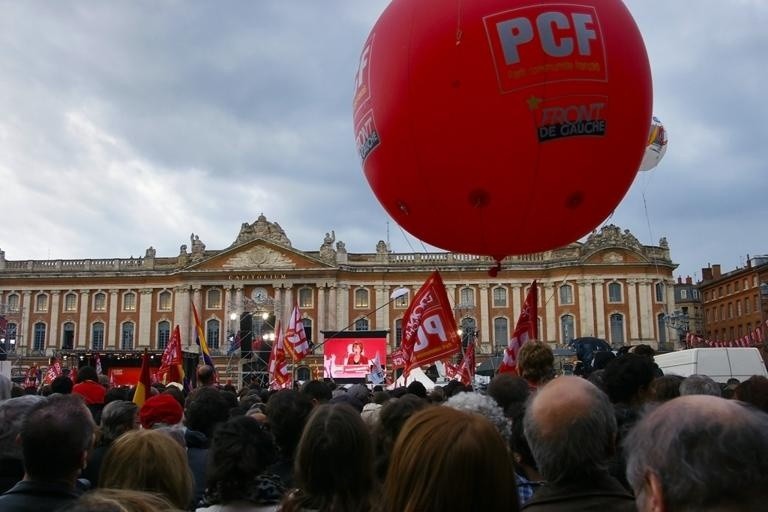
[139,394,183,429]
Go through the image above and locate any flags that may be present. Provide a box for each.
[154,325,184,384]
[496,277,542,390]
[191,301,216,375]
[269,319,291,390]
[283,300,310,362]
[226,329,244,356]
[400,269,462,382]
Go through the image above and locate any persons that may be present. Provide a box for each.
[587,228,638,246]
[0,338,768,511]
[324,230,336,245]
[190,233,206,258]
